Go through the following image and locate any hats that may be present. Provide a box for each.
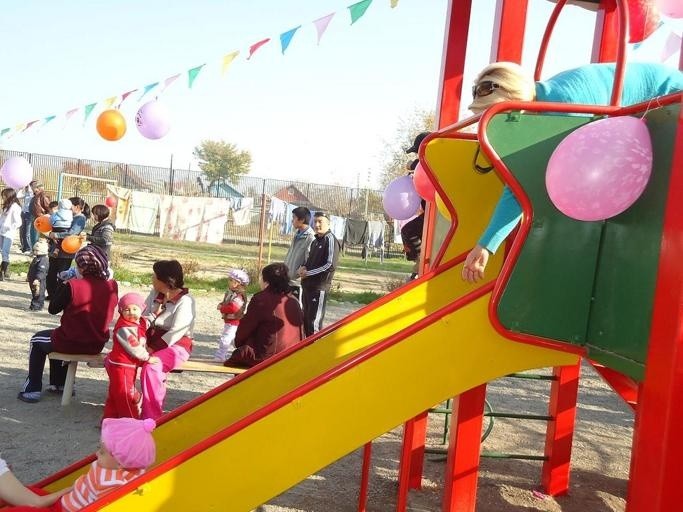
[118,293,146,313]
[229,268,249,285]
[102,417,156,470]
[406,132,431,153]
[58,199,72,209]
[76,245,109,280]
[34,240,47,251]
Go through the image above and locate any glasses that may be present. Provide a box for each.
[472,81,501,96]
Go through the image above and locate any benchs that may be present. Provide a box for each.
[49,348,250,405]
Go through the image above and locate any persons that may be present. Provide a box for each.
[297,212,341,338]
[0,188,22,280]
[78,205,114,258]
[0,458,74,507]
[461,62,683,285]
[140,260,195,420]
[284,207,315,340]
[214,269,250,362]
[45,196,90,300]
[18,244,118,403]
[401,132,433,283]
[26,238,49,314]
[224,263,305,369]
[16,180,37,254]
[59,267,113,280]
[101,294,166,426]
[49,199,72,256]
[0,418,156,512]
[34,200,58,240]
[28,182,51,257]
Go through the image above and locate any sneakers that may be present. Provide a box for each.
[47,384,76,396]
[18,392,41,403]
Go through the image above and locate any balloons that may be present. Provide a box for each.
[628,1,660,43]
[657,0,683,20]
[35,216,52,233]
[413,161,435,202]
[545,110,654,222]
[383,175,420,220]
[436,190,452,222]
[96,109,128,141]
[0,157,33,189]
[106,196,118,208]
[61,236,82,253]
[135,96,171,139]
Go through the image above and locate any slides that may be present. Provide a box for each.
[0,93,683,512]
